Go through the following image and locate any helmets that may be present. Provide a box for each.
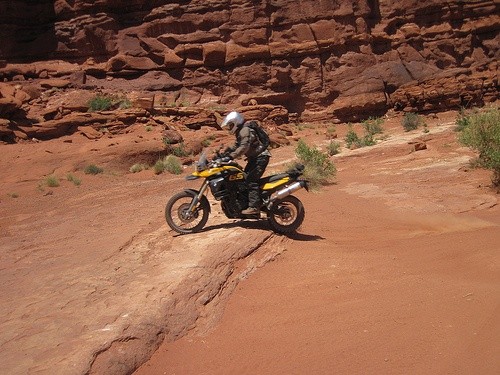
[220,111,245,135]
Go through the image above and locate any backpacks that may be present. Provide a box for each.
[243,120,271,161]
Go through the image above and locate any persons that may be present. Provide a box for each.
[213,111,271,216]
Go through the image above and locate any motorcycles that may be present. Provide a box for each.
[164,143,310,235]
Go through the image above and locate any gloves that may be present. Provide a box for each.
[219,152,229,157]
[218,154,230,162]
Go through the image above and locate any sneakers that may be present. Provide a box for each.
[241,206,260,214]
